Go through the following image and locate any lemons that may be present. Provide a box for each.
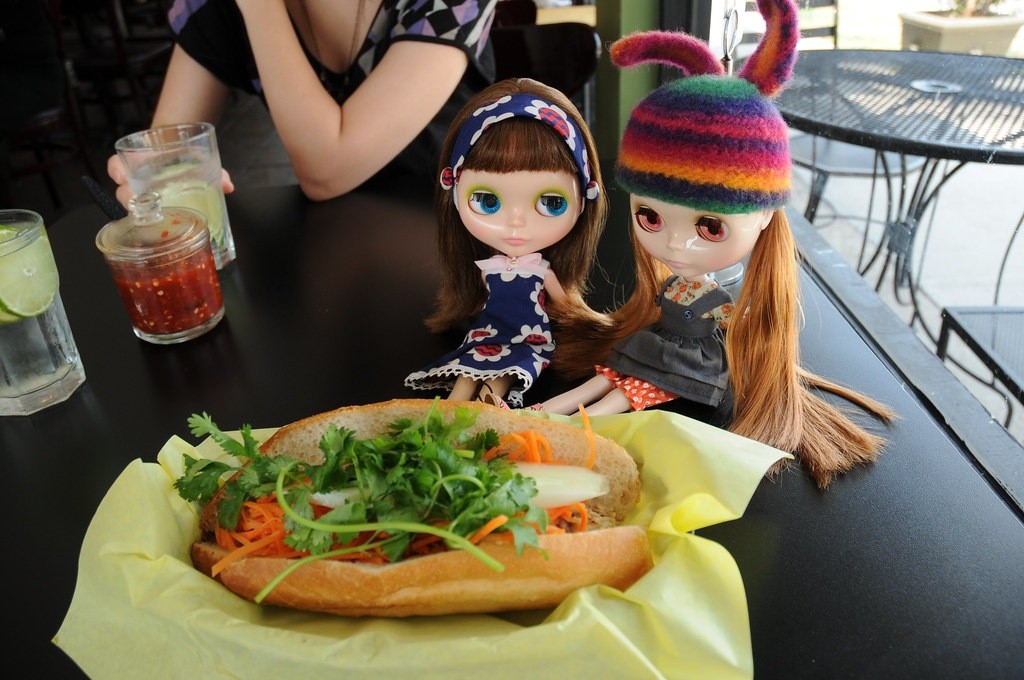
[153,180,225,247]
[0,224,61,324]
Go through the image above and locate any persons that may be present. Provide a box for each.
[484,0,894,489]
[109,0,498,216]
[404,77,605,405]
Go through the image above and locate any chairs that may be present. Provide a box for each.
[0,0,175,213]
[935,305,1024,424]
[741,0,927,225]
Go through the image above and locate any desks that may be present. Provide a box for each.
[0,156,1024,679]
[734,50,1024,428]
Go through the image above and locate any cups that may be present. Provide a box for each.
[0,209,87,416]
[114,121,236,271]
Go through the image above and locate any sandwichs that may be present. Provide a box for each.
[173,397,652,617]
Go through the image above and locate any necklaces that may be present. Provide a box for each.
[299,0,364,104]
